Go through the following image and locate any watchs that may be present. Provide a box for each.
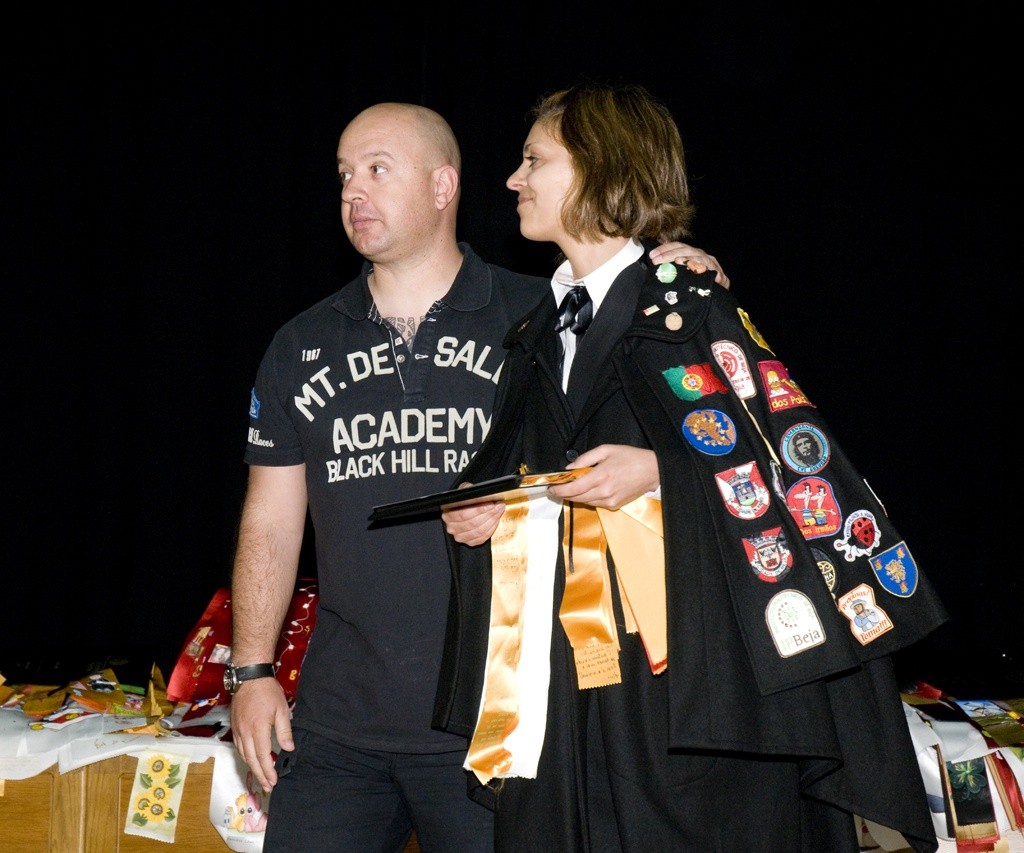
[223,662,276,696]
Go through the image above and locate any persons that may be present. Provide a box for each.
[431,84,946,852]
[228,104,729,852]
